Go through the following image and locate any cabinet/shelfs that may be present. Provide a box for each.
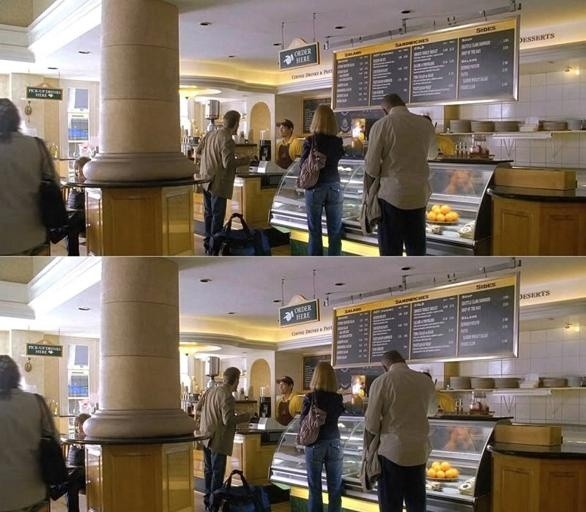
[267,155,511,258]
[267,411,508,512]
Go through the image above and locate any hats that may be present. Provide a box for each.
[275,118,294,128]
[276,376,293,385]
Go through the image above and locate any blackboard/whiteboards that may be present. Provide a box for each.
[331,14,521,113]
[331,272,521,369]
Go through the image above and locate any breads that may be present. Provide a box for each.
[427,460,458,479]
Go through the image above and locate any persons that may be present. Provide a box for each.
[363,350,435,512]
[274,118,300,169]
[194,110,253,257]
[51,157,92,256]
[299,105,345,256]
[364,93,438,256]
[274,376,305,426]
[300,361,345,512]
[0,98,59,257]
[194,367,251,511]
[1,355,65,511]
[50,414,92,512]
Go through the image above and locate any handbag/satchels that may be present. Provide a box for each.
[208,212,271,256]
[34,135,70,243]
[297,137,326,189]
[296,391,327,445]
[34,393,68,502]
[207,469,271,512]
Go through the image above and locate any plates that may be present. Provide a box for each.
[495,121,519,129]
[497,376,521,389]
[471,122,493,132]
[540,376,566,387]
[425,220,460,226]
[471,377,494,388]
[449,120,469,131]
[542,120,565,132]
[426,476,459,482]
[449,376,469,389]
